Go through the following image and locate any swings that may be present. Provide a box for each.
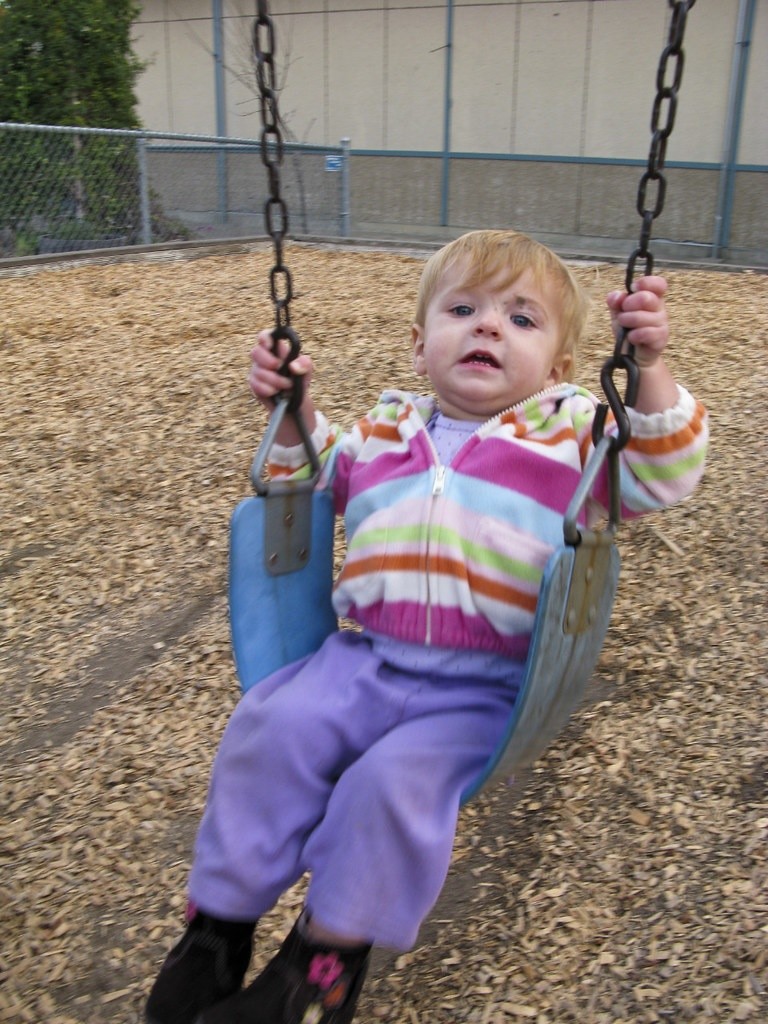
[224,2,688,812]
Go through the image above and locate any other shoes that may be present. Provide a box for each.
[144,908,257,1024]
[197,908,374,1024]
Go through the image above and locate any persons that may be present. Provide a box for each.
[143,232,710,1023]
